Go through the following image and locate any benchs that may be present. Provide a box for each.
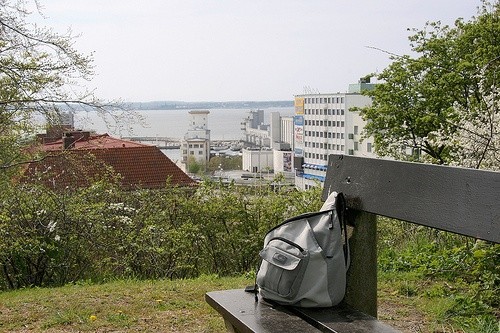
[205,152,500,333]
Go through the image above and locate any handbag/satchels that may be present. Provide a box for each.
[246,190,352,309]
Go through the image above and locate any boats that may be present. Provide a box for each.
[228,138,244,151]
[210,140,231,151]
